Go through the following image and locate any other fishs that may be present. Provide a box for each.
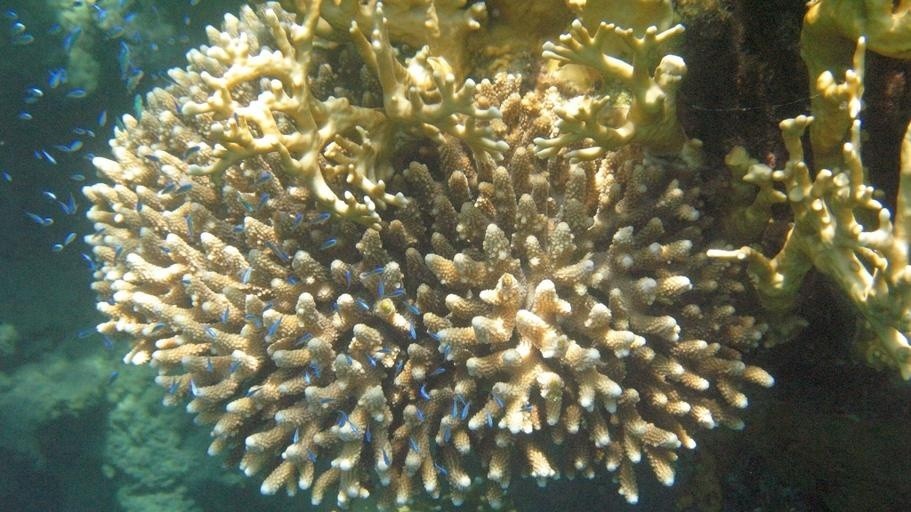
[0,1,533,479]
